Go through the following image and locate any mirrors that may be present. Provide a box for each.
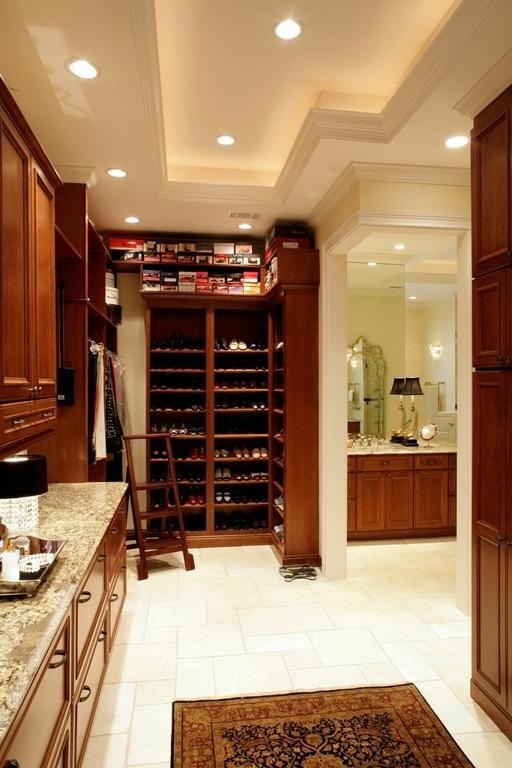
[348,263,461,448]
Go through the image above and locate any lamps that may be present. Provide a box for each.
[404,377,424,446]
[389,377,411,443]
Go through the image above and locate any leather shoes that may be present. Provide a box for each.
[151,355,268,370]
[151,509,268,532]
[151,484,267,507]
[150,413,267,436]
[150,374,268,389]
[150,438,268,459]
[150,392,267,412]
[150,461,269,483]
[151,332,284,351]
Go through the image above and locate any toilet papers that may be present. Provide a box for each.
[0,551,20,581]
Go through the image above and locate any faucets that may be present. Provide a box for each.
[357,433,365,448]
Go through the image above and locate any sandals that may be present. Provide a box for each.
[215,257,258,264]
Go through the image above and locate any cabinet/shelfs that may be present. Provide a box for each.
[1,76,320,581]
[348,455,457,545]
[0,478,139,766]
[470,85,510,745]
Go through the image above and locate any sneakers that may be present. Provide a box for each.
[274,523,284,542]
[273,494,284,512]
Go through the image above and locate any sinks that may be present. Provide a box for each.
[358,446,387,452]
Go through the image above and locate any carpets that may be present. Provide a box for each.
[171,682,478,768]
